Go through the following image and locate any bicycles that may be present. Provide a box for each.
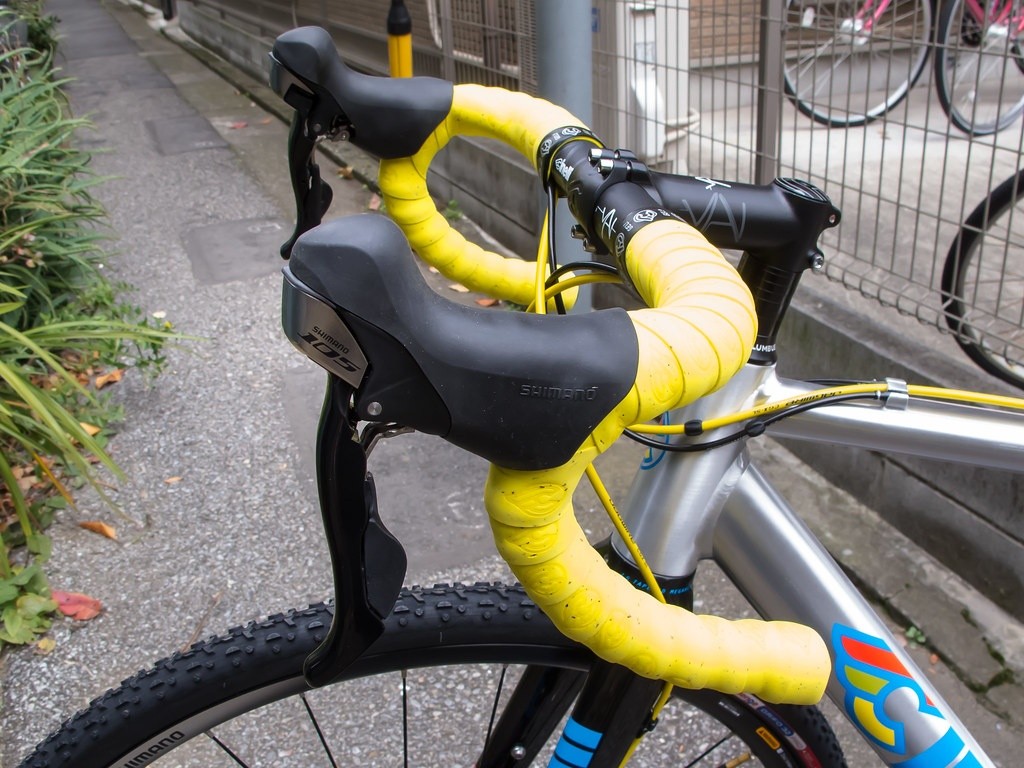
[20,26,1024,767]
[939,165,1024,388]
[932,2,1024,137]
[780,0,936,130]
